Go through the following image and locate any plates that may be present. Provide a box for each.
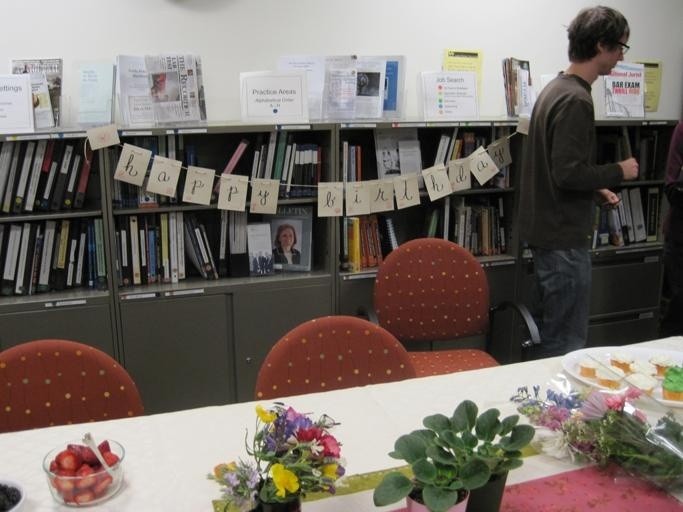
[562,345,683,411]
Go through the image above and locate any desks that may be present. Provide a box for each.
[0,335,683,511]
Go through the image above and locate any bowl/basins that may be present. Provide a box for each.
[42,435,126,508]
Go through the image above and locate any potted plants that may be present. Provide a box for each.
[373,400,535,510]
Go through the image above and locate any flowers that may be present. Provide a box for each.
[213,384,345,511]
[508,379,683,504]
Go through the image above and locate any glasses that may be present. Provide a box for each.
[619,40,629,53]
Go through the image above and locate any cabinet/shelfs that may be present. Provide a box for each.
[340,122,520,366]
[1,118,120,369]
[588,122,674,328]
[106,124,336,413]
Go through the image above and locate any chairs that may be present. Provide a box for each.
[0,340,142,436]
[255,317,417,399]
[359,239,542,379]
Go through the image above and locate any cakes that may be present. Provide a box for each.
[579,350,683,401]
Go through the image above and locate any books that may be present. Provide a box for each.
[597,126,668,250]
[1,142,94,216]
[3,218,109,298]
[110,130,321,210]
[342,123,511,273]
[115,207,312,288]
[501,57,533,117]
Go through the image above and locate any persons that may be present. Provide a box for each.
[517,5,641,358]
[272,224,301,265]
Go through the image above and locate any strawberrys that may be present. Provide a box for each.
[50,439,121,502]
